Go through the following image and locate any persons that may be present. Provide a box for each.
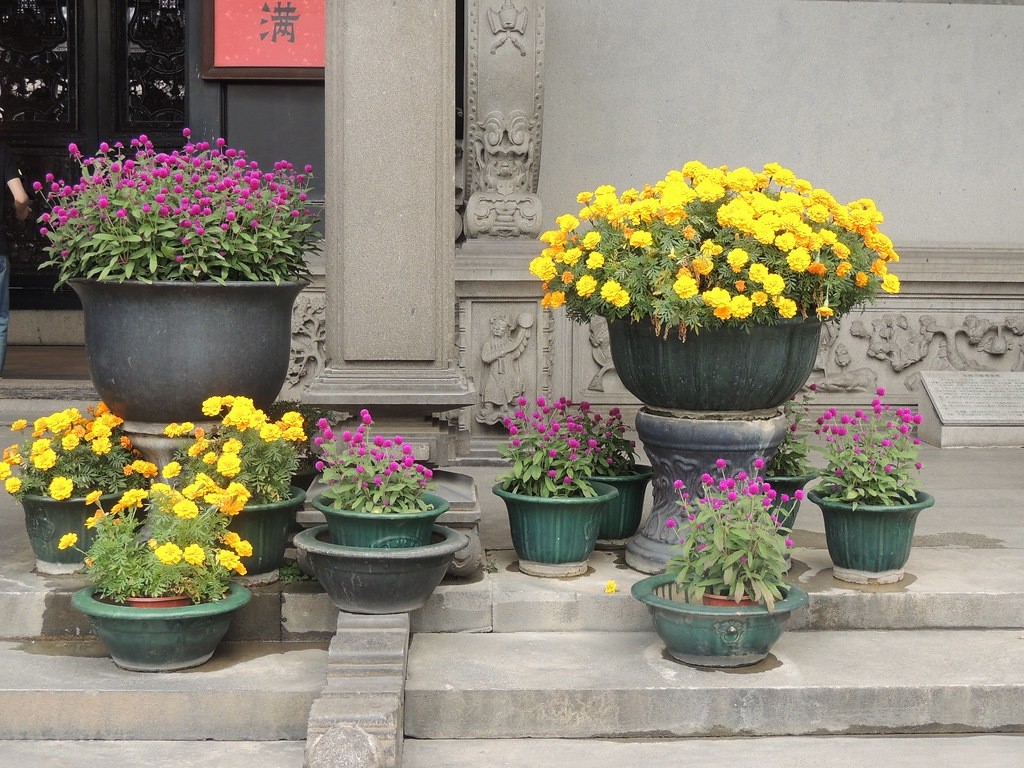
[0,149,33,376]
[480,314,529,418]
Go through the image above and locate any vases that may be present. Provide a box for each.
[65,277,309,424]
[579,464,656,539]
[807,484,935,572]
[199,484,306,574]
[125,594,191,607]
[491,481,620,565]
[595,310,833,410]
[71,585,253,671]
[702,593,756,606]
[310,492,449,548]
[293,526,470,616]
[18,492,123,563]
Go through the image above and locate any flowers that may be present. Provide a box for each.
[57,459,253,595]
[668,457,804,614]
[503,395,642,496]
[528,160,901,342]
[813,386,927,511]
[315,409,435,512]
[0,401,158,502]
[165,395,308,500]
[33,128,326,294]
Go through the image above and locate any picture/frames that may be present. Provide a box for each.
[200,0,325,80]
